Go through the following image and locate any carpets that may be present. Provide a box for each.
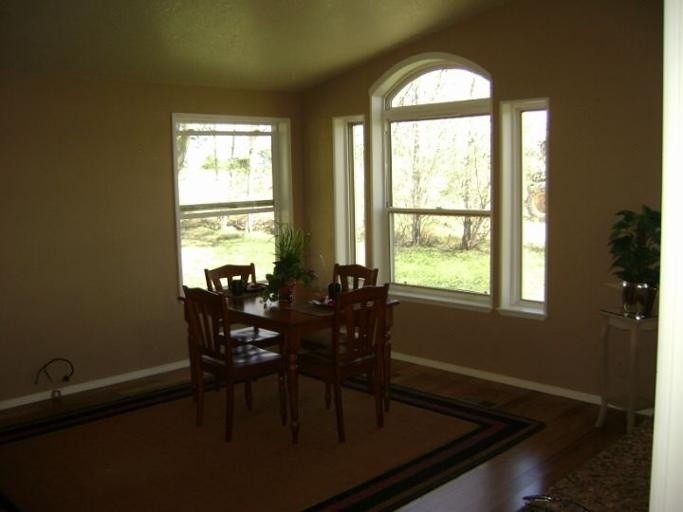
[0,359,544,512]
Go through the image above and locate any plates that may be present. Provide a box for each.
[308,296,332,305]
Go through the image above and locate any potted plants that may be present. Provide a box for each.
[257,219,320,311]
[605,205,661,316]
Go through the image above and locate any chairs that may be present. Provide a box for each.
[182,263,400,444]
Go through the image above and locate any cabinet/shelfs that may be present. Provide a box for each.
[593,305,659,434]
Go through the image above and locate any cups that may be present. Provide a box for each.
[328,283,340,300]
[228,281,242,297]
